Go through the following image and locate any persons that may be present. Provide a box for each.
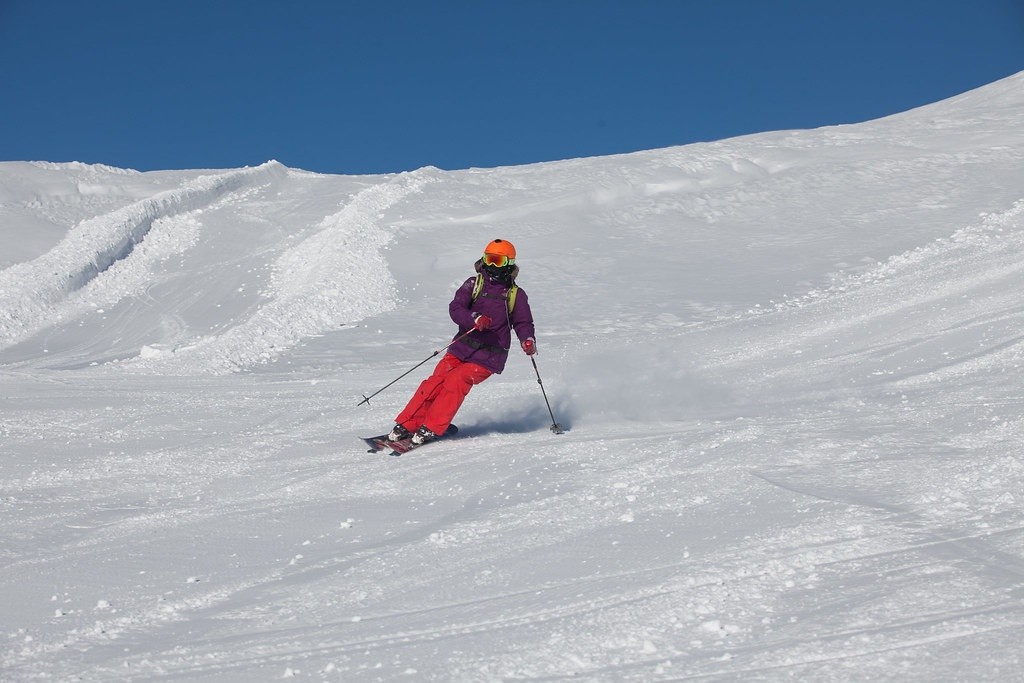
[387,239,537,446]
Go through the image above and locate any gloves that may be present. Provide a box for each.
[475,315,492,331]
[522,337,536,355]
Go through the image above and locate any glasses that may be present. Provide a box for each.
[483,254,516,267]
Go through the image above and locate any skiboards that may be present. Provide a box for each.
[361,423,458,456]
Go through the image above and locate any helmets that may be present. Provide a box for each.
[484,239,516,258]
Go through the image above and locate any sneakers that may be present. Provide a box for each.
[389,425,408,441]
[412,425,432,445]
